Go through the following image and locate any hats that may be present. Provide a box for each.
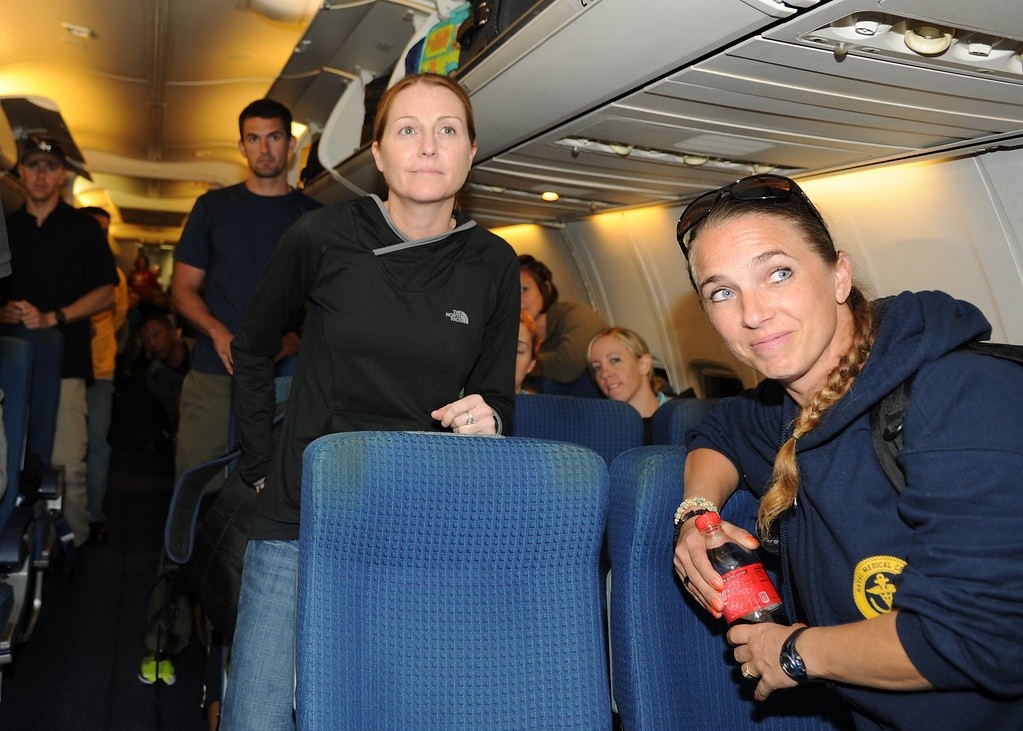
[19,138,66,166]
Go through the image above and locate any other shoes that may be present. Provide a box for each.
[88,522,108,545]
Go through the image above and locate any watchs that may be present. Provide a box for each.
[54,309,67,324]
[779,626,813,684]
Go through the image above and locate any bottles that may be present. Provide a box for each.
[694,511,791,631]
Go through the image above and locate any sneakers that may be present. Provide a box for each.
[139,642,177,685]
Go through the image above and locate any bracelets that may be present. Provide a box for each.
[674,496,719,530]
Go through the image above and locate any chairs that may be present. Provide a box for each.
[651,398,722,447]
[526,362,605,400]
[299,433,615,731]
[25,332,62,573]
[507,392,645,460]
[0,336,41,669]
[606,443,839,731]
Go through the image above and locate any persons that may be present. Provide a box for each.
[0,134,197,574]
[515,254,609,395]
[138,72,522,731]
[673,175,1023,731]
[587,327,676,446]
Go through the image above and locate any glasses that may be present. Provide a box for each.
[676,174,834,294]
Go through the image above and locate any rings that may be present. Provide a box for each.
[684,576,690,587]
[466,411,476,425]
[743,663,760,678]
